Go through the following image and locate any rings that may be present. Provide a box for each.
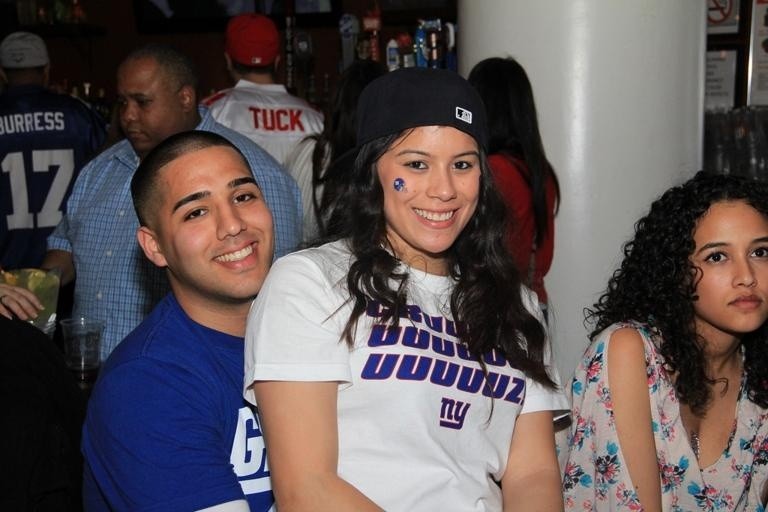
[0,292,9,304]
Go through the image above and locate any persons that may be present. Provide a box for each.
[242,67,573,512]
[83,129,278,512]
[0,32,106,271]
[198,15,331,246]
[467,56,561,325]
[560,170,768,512]
[42,45,305,373]
[0,282,47,322]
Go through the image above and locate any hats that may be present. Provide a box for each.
[0,32,49,68]
[328,68,490,166]
[224,13,284,66]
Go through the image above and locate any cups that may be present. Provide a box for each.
[60,319,106,379]
[1,269,59,339]
[707,105,767,182]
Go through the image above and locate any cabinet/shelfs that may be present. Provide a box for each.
[281,0,459,105]
